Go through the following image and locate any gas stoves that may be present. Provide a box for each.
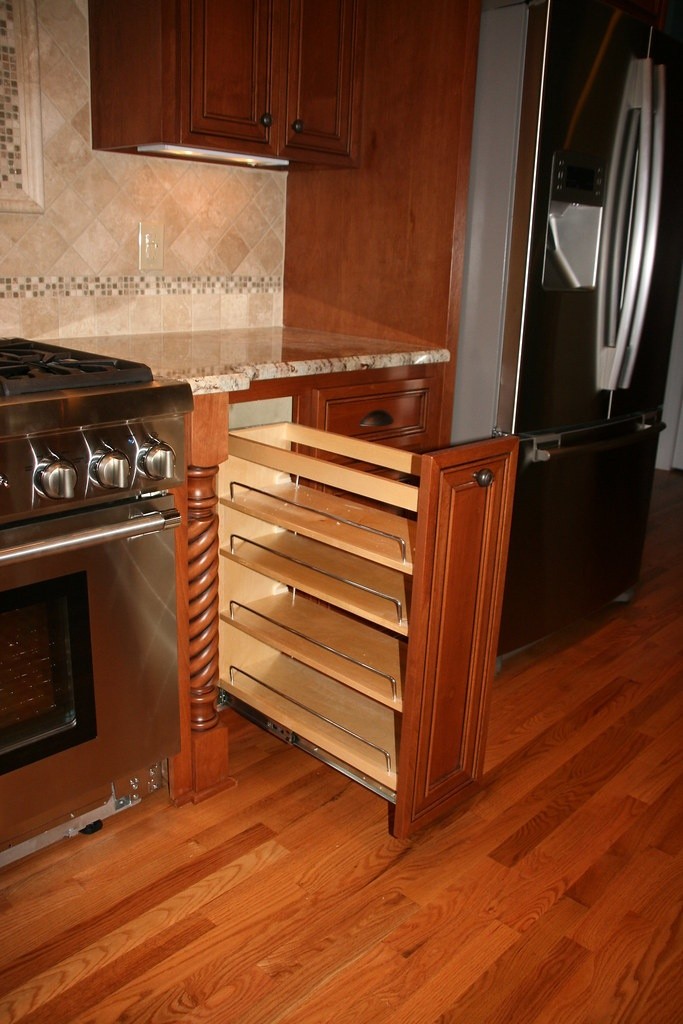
[0,337,194,508]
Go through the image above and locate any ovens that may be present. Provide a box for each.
[2,493,193,873]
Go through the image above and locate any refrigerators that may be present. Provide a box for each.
[450,0,683,659]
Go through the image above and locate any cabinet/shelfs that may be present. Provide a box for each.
[87,0,370,171]
[217,419,521,842]
[227,360,447,642]
